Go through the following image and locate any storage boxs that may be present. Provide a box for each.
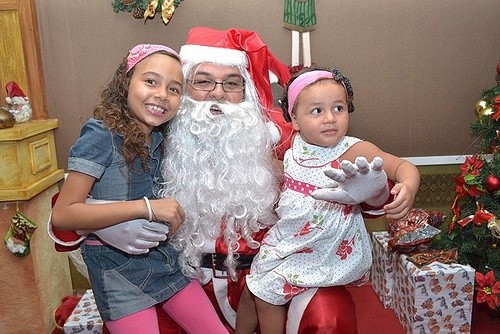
[64,289,104,334]
[371,230,476,334]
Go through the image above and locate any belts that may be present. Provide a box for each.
[198,252,257,279]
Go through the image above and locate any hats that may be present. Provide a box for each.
[178,27,291,113]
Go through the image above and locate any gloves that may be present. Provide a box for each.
[310,156,390,206]
[74,195,169,255]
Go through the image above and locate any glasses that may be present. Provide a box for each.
[186,75,245,92]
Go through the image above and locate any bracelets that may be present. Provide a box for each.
[143,196,157,222]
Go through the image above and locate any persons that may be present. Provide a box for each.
[5,81,33,123]
[47,27,401,334]
[236,63,421,334]
[51,43,231,334]
